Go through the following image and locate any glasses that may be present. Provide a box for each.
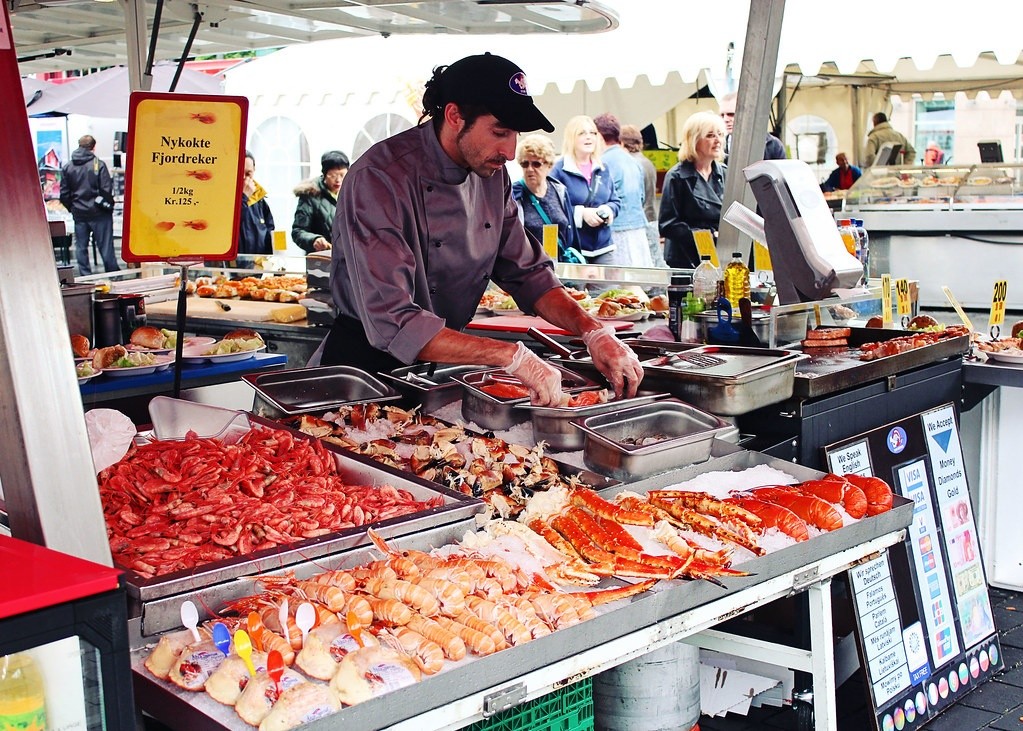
[519,160,547,168]
[326,174,346,180]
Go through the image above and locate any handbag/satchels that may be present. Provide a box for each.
[94,190,115,212]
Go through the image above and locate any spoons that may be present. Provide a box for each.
[180,600,231,658]
[267,650,284,696]
[233,612,263,676]
[346,610,365,648]
[279,598,320,649]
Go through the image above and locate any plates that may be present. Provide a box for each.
[168,344,267,363]
[124,336,216,355]
[588,306,655,321]
[654,310,669,316]
[77,369,103,385]
[100,357,176,375]
[74,357,93,360]
[983,348,1023,363]
[475,302,525,315]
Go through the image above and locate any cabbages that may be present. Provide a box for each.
[77,329,262,378]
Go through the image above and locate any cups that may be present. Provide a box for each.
[681,317,704,340]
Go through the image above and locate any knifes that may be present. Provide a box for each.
[216,299,231,311]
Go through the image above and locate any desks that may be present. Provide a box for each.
[73,354,288,406]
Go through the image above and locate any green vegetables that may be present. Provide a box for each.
[496,289,647,316]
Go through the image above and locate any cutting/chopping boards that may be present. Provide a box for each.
[145,297,301,322]
[707,297,791,349]
[466,315,634,336]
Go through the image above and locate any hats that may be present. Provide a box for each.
[441,51,555,133]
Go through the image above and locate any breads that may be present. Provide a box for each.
[184,276,307,303]
[71,333,90,358]
[224,329,264,343]
[92,344,126,368]
[648,294,670,311]
[130,326,166,349]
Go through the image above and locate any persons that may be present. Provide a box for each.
[925,140,938,165]
[658,112,727,268]
[549,115,622,298]
[865,112,916,165]
[619,125,671,297]
[203,150,276,279]
[291,150,350,255]
[824,153,863,189]
[510,134,582,262]
[593,114,655,302]
[306,51,644,408]
[59,135,123,282]
[719,91,786,165]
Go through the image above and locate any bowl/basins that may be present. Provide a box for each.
[750,288,770,304]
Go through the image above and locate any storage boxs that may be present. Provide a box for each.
[456,675,594,731]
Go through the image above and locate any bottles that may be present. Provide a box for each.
[693,256,719,304]
[667,276,694,342]
[725,253,751,317]
[0,654,46,731]
[837,218,869,286]
